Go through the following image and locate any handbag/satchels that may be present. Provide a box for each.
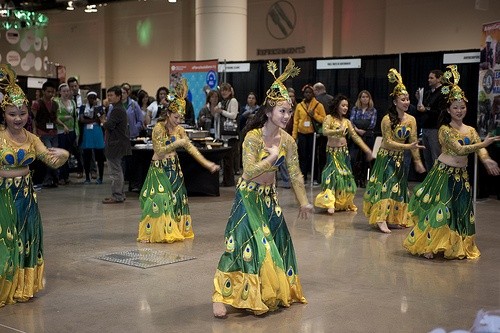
[311,118,321,133]
[224,119,238,132]
[352,118,372,129]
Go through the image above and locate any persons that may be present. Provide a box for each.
[312,81,338,185]
[478,34,495,71]
[479,97,493,136]
[362,68,425,234]
[290,83,327,185]
[1,60,301,204]
[212,55,315,318]
[402,64,499,261]
[136,76,222,243]
[413,69,453,182]
[347,90,380,188]
[1,65,70,309]
[312,91,376,216]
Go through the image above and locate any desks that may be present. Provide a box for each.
[130,124,233,196]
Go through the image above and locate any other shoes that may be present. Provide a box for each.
[33,167,104,188]
[314,179,320,187]
[232,169,241,175]
[102,195,126,204]
